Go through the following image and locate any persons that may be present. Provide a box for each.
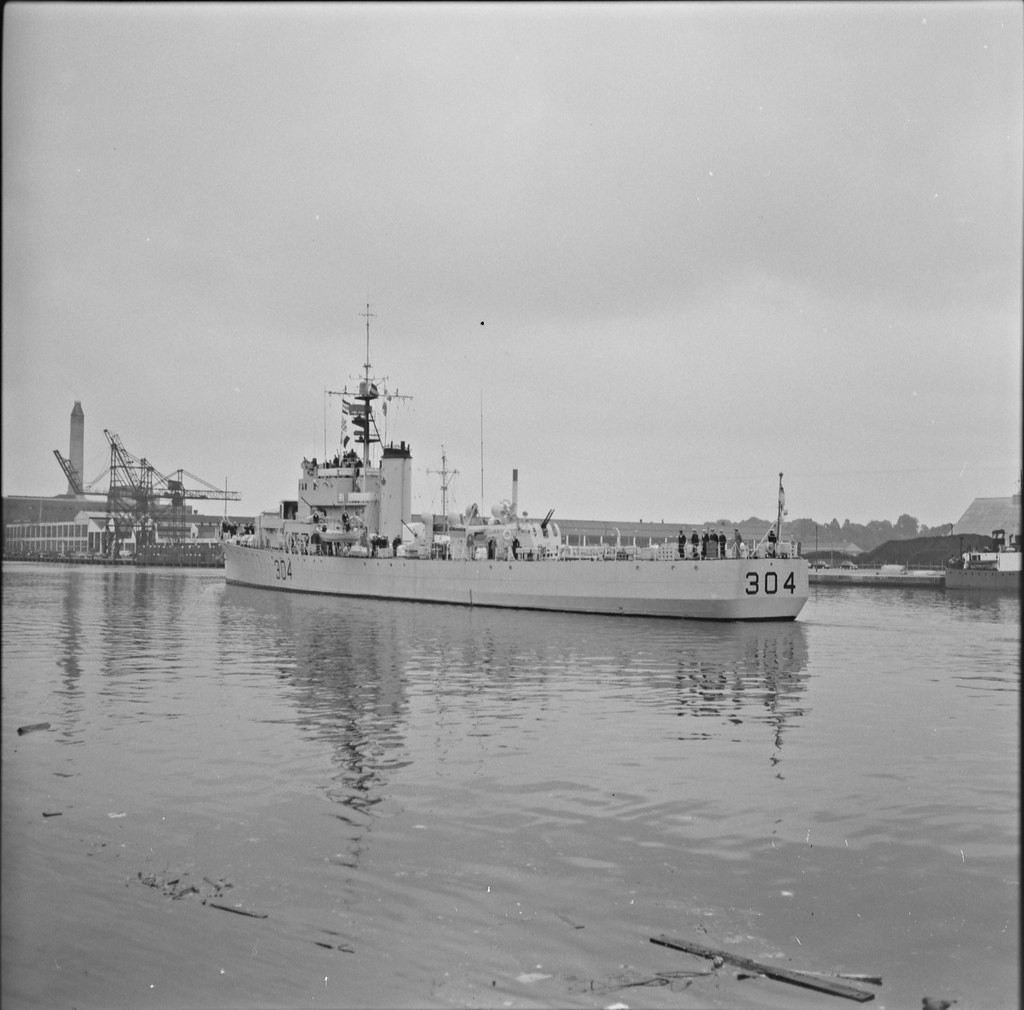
[734,528,742,559]
[709,528,719,556]
[768,530,778,558]
[322,523,327,532]
[346,523,351,531]
[303,447,365,468]
[313,512,319,523]
[677,530,687,557]
[718,530,727,558]
[488,536,497,558]
[342,510,350,524]
[511,535,520,560]
[221,520,255,537]
[370,534,402,557]
[691,529,700,558]
[701,528,708,559]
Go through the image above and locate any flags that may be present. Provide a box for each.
[779,484,786,513]
[339,379,393,449]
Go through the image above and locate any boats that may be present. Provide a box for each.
[942,529,1022,591]
[225,300,809,622]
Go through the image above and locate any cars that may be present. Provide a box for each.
[838,560,858,570]
[811,560,831,569]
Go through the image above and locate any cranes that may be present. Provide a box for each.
[53,428,241,563]
